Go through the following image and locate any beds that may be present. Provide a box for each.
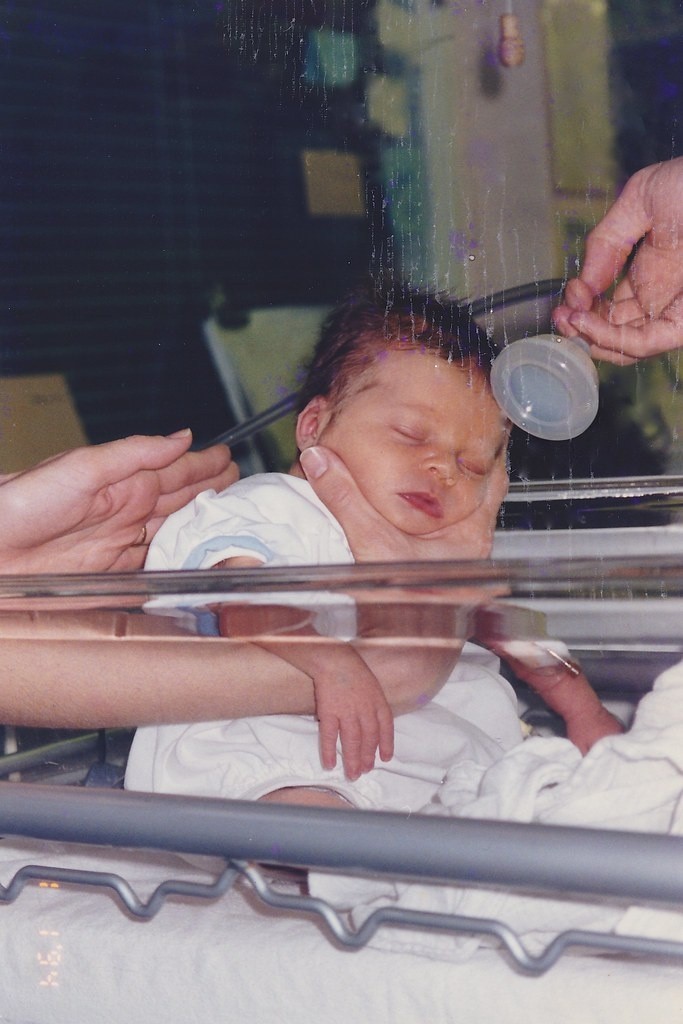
[0,475,683,1023]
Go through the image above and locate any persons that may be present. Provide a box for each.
[124,285,630,811]
[0,424,509,731]
[551,153,682,367]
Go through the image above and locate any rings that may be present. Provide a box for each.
[134,526,148,546]
